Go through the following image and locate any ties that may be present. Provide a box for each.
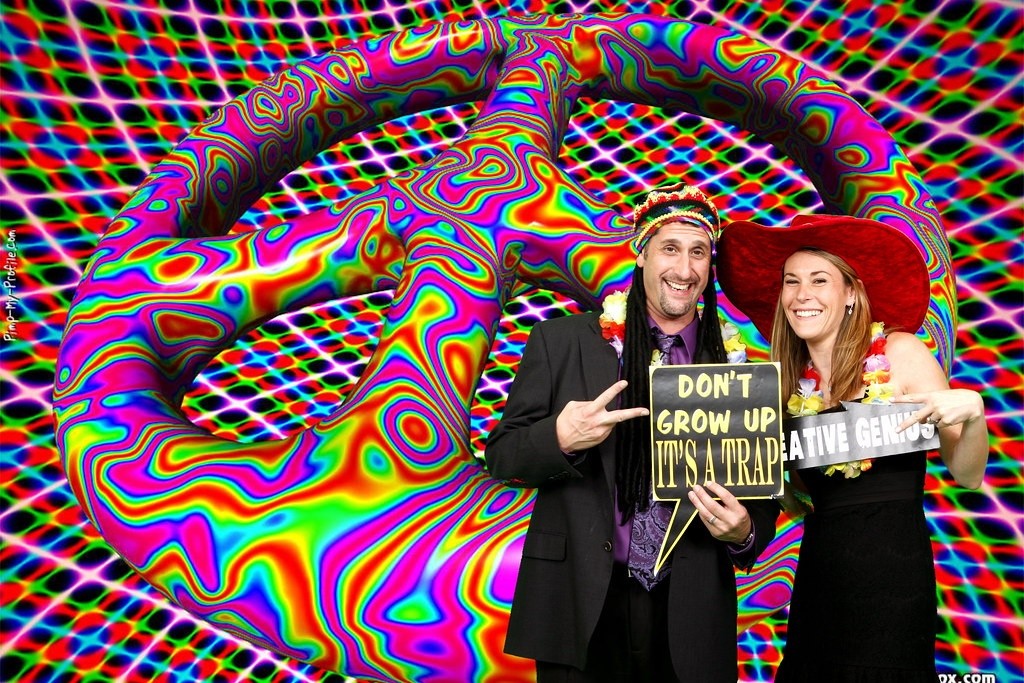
[628,332,676,592]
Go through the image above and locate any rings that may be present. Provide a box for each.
[710,516,717,524]
[927,417,943,425]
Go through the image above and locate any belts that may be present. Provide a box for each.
[614,564,667,578]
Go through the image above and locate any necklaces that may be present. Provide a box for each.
[787,321,892,481]
[596,287,748,383]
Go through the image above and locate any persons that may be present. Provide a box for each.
[717,214,989,683]
[489,184,783,683]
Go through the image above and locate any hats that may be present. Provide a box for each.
[630,181,721,260]
[714,215,931,346]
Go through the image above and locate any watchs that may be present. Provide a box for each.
[738,533,753,547]
